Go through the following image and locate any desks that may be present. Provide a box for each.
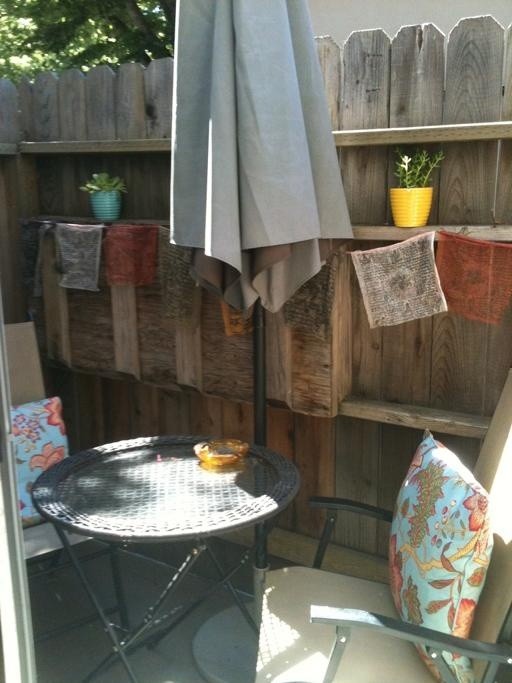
[31,431,302,683]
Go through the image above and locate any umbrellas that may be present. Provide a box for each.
[171,0,354,627]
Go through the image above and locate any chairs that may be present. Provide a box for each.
[253,368,512,683]
[4,319,132,646]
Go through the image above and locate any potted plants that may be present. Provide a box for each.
[77,171,129,222]
[389,147,447,228]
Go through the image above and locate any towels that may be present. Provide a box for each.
[15,219,56,319]
[283,249,341,335]
[350,229,449,330]
[151,226,196,305]
[52,220,107,294]
[437,230,511,325]
[103,222,160,288]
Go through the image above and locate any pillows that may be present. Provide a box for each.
[9,394,71,531]
[387,428,494,683]
